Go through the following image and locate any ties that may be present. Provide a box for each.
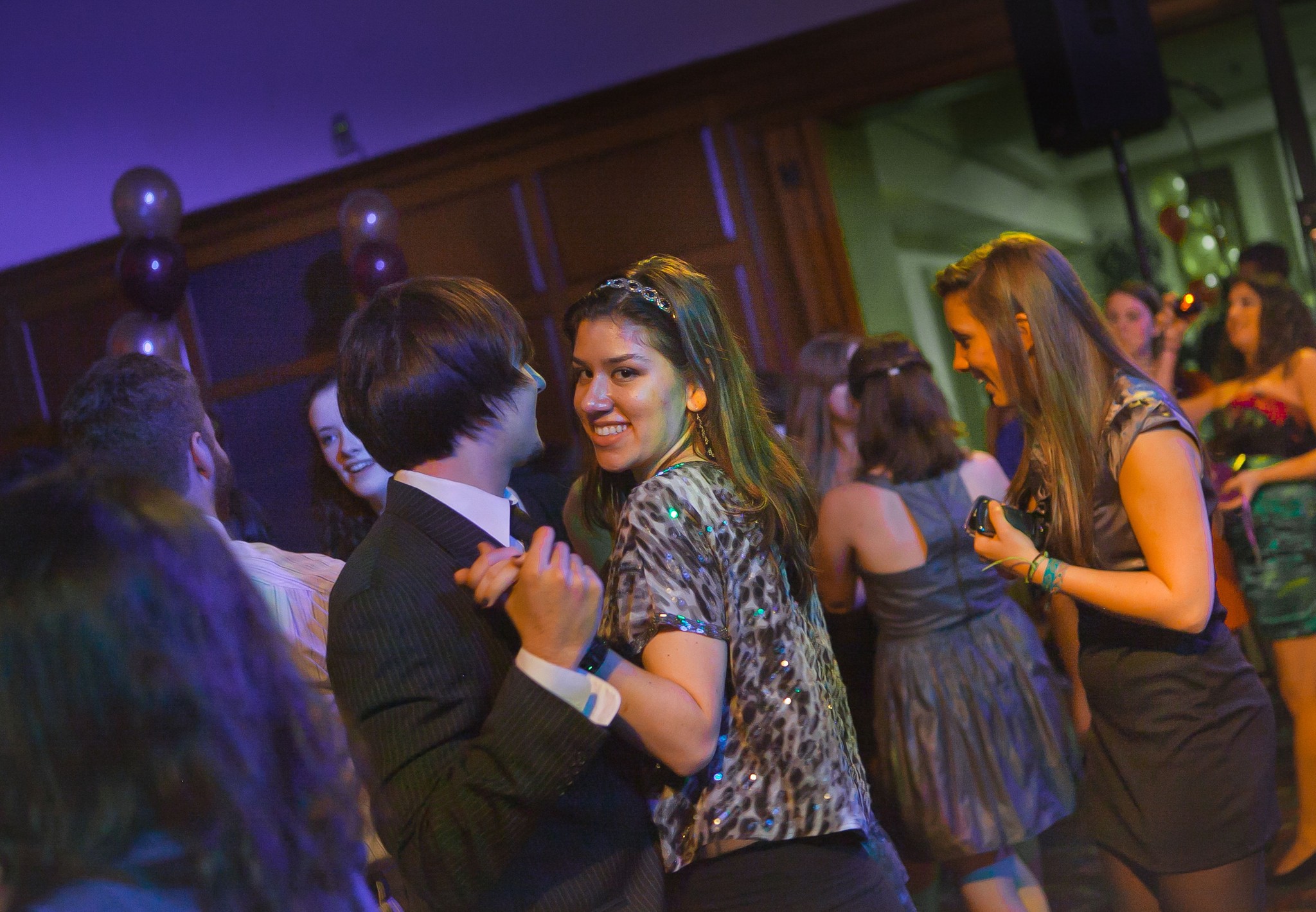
[510,503,538,553]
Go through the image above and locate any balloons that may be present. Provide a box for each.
[106,164,410,367]
[1152,159,1250,321]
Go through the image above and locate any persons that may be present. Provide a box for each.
[934,227,1276,912]
[46,352,408,909]
[322,276,679,912]
[303,366,397,526]
[815,336,1088,912]
[561,456,636,581]
[452,256,915,908]
[1141,269,1316,891]
[773,335,939,912]
[1,467,376,912]
[979,229,1309,486]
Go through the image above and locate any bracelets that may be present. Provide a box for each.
[1021,552,1070,594]
[575,634,610,673]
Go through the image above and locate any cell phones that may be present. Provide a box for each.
[969,497,1048,552]
